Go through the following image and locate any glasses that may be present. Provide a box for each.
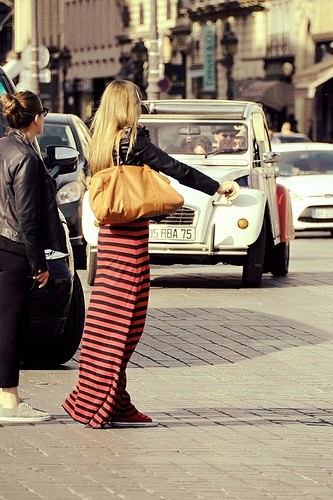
[37,107,49,117]
[222,134,235,138]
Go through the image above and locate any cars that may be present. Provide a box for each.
[33,112,94,271]
[82,99,294,287]
[270,132,316,163]
[269,142,333,238]
[0,65,86,367]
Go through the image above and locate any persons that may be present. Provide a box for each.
[62,82,235,428]
[1,91,61,422]
[175,117,252,155]
[295,155,327,172]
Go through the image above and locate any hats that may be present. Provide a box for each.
[211,125,240,135]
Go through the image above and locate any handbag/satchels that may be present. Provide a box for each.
[89,130,184,224]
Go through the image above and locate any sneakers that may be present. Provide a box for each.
[0,400,51,423]
[109,412,159,427]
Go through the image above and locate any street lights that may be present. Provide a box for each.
[59,43,73,115]
[132,36,150,101]
[220,21,240,102]
